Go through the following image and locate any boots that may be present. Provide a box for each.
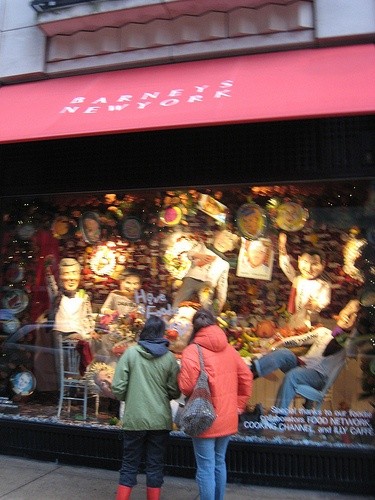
[116,484,132,500]
[146,486,161,500]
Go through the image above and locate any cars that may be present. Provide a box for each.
[0,322,125,405]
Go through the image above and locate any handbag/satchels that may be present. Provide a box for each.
[174,344,217,438]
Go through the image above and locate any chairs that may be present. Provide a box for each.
[57,334,100,421]
[268,361,346,436]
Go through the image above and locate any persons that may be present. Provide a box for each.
[171,228,239,316]
[43,257,101,405]
[177,308,254,500]
[99,268,144,319]
[243,298,363,428]
[109,316,183,500]
[278,230,332,326]
